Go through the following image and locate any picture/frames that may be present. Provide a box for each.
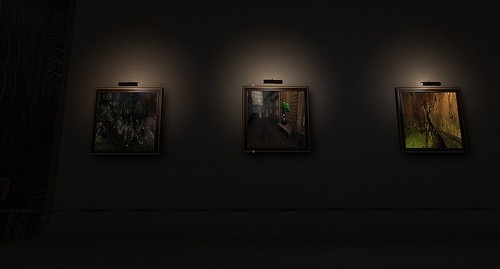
[394,86,470,155]
[241,83,312,154]
[88,86,164,158]
